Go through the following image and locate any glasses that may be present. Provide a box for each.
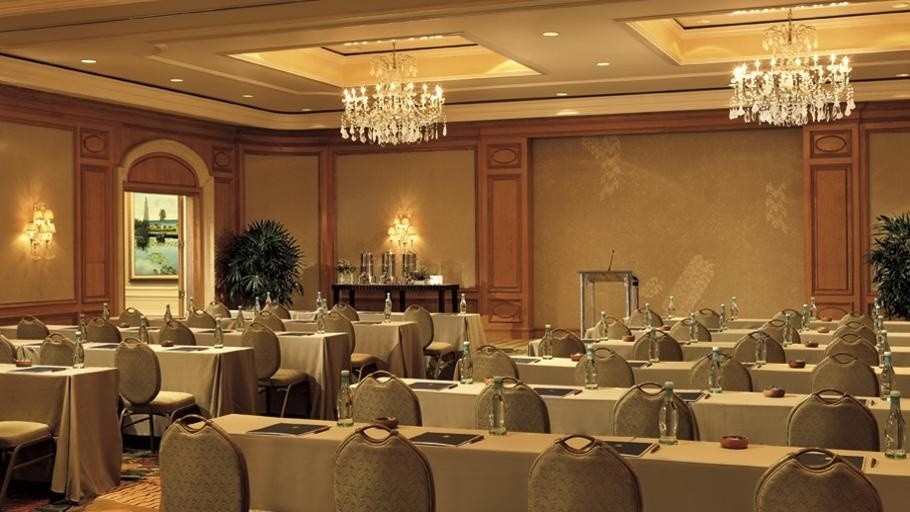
[729,5,857,124]
[343,40,447,146]
[387,215,419,243]
[26,204,57,259]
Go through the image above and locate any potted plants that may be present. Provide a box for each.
[125,192,179,280]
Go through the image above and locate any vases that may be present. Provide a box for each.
[411,266,427,280]
[336,257,356,273]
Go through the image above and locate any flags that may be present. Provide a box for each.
[338,273,353,285]
[413,281,425,286]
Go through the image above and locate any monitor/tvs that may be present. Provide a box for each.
[797,453,864,471]
[133,328,160,330]
[673,392,705,401]
[29,343,41,345]
[93,344,119,348]
[67,327,77,329]
[603,441,654,457]
[823,397,869,405]
[582,340,594,344]
[198,331,232,334]
[293,321,316,323]
[169,347,209,352]
[15,367,66,372]
[510,358,540,364]
[626,362,646,368]
[280,332,313,336]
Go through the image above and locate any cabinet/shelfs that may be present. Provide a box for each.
[73,332,85,369]
[874,306,881,315]
[708,346,723,393]
[802,304,809,330]
[648,332,659,364]
[689,312,698,343]
[489,377,508,435]
[460,293,466,313]
[255,297,260,318]
[542,324,553,359]
[599,311,608,339]
[461,342,473,384]
[215,318,223,348]
[783,314,792,345]
[878,352,897,399]
[809,298,816,320]
[139,315,148,345]
[189,297,194,312]
[336,370,354,428]
[755,332,768,364]
[873,298,880,307]
[644,303,651,328]
[659,382,679,445]
[79,313,87,342]
[876,330,893,368]
[316,292,321,308]
[730,297,738,319]
[103,302,109,322]
[719,303,728,331]
[235,306,244,332]
[883,391,907,458]
[586,345,599,390]
[164,305,172,324]
[265,292,270,304]
[318,309,324,334]
[667,296,675,318]
[322,299,328,316]
[385,293,392,321]
[875,316,883,332]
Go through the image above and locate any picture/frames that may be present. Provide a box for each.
[579,269,639,340]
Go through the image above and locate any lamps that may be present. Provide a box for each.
[733,332,786,363]
[833,320,876,346]
[669,317,712,342]
[240,321,312,417]
[159,321,196,344]
[159,415,249,512]
[827,333,879,366]
[263,302,290,318]
[754,449,882,512]
[808,354,877,396]
[351,370,421,427]
[786,390,879,449]
[538,327,585,357]
[817,307,846,320]
[758,319,800,344]
[332,425,435,512]
[404,305,456,379]
[614,383,698,440]
[40,332,76,366]
[0,335,17,365]
[527,433,642,511]
[781,309,805,329]
[330,302,360,321]
[688,350,752,392]
[0,420,56,499]
[320,311,377,379]
[86,317,120,342]
[454,345,520,383]
[572,348,634,387]
[114,338,200,455]
[120,307,148,327]
[632,329,682,361]
[187,309,215,328]
[17,315,49,340]
[476,376,549,436]
[628,304,664,329]
[691,308,721,329]
[254,310,284,332]
[206,301,231,318]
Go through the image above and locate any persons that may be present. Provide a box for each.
[248,423,329,436]
[408,383,453,390]
[534,388,575,396]
[742,364,755,368]
[408,432,478,447]
[354,322,381,324]
